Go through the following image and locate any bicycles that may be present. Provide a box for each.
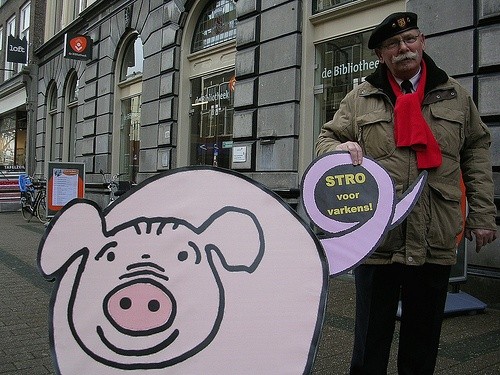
[19,177,52,224]
[99,169,127,208]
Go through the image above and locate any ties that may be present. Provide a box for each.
[399,81,413,94]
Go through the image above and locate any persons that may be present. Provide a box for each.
[314,11,500,374]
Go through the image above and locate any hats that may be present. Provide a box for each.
[365,11,419,50]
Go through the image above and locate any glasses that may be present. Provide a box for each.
[380,34,421,50]
